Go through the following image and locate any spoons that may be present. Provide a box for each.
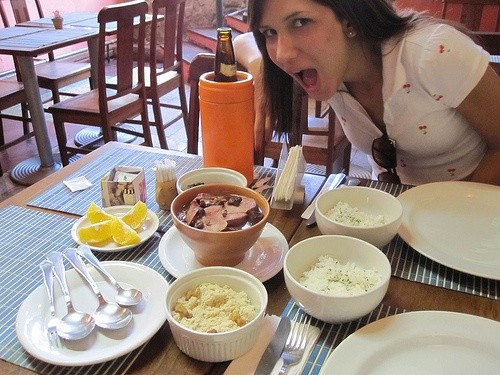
[46,251,95,342]
[75,243,144,307]
[61,247,133,329]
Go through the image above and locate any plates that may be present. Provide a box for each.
[318,310,499,375]
[396,180,500,279]
[70,204,160,253]
[158,222,290,282]
[14,260,169,367]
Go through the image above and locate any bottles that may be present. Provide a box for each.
[213,27,238,82]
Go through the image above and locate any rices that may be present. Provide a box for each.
[299,256,381,295]
[323,201,385,227]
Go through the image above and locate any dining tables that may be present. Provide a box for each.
[208,174,500,375]
[0,140,326,375]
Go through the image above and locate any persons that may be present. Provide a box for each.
[233,0,500,186]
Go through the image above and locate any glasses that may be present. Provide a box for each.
[371,132,401,184]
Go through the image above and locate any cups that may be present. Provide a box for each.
[198,71,254,188]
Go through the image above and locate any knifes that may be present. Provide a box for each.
[306,179,361,226]
[253,316,291,374]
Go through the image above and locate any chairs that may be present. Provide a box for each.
[0,0,352,176]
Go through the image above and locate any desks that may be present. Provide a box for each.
[0,12,99,186]
[14,11,164,149]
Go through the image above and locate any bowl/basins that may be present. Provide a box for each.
[282,234,392,325]
[315,186,404,249]
[164,265,269,362]
[175,167,248,196]
[169,184,270,266]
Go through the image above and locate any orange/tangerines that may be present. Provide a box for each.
[77,201,148,246]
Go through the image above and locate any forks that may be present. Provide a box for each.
[38,260,63,348]
[278,313,312,374]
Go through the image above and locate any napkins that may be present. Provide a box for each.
[63,176,93,192]
[222,313,320,375]
